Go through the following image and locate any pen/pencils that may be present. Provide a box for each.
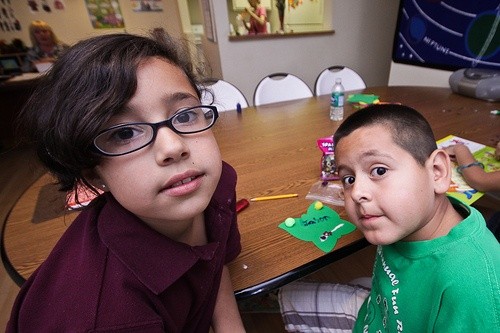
[250,193,299,202]
[490,110,499,114]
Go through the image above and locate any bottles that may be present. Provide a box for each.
[329,78,344,121]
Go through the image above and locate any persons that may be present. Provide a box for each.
[443,142,500,192]
[4,34,246,333]
[241,0,268,36]
[278,104,500,333]
[26,21,70,73]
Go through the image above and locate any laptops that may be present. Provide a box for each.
[0,56,26,80]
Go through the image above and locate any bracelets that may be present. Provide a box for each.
[457,162,485,174]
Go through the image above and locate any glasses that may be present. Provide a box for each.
[86,105,220,159]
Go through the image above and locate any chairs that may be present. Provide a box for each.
[314,65,367,96]
[197,78,248,113]
[252,72,314,105]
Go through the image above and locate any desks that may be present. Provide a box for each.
[0,87,499,302]
